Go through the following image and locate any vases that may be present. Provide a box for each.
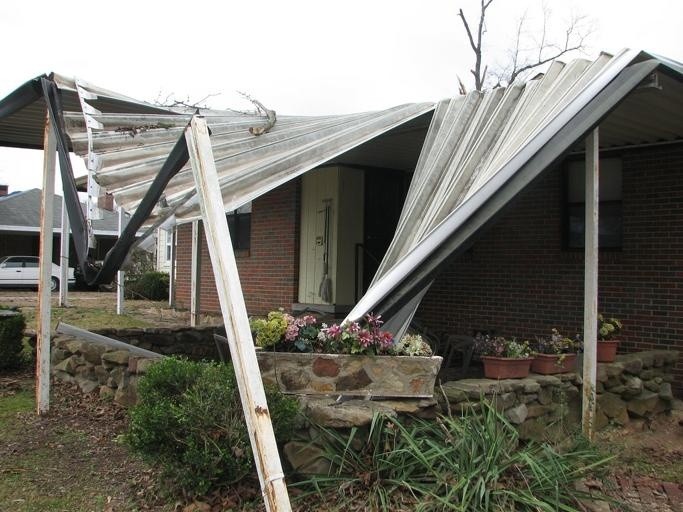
[597,339,618,363]
[530,352,576,375]
[255,350,445,400]
[481,355,535,380]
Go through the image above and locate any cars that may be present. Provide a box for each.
[0,256,76,293]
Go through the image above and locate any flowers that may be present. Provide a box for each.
[472,335,532,360]
[530,329,584,367]
[597,314,623,341]
[253,309,433,358]
